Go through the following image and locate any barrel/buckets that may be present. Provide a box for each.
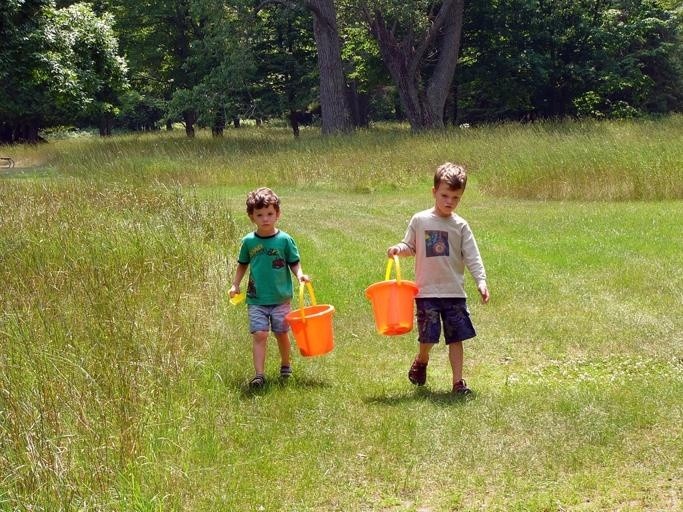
[365,255,418,336]
[286,279,336,357]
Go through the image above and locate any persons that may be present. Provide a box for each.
[388,162,490,396]
[228,188,310,388]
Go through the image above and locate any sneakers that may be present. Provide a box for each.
[250,377,265,385]
[407,357,430,386]
[281,364,291,376]
[452,379,472,394]
